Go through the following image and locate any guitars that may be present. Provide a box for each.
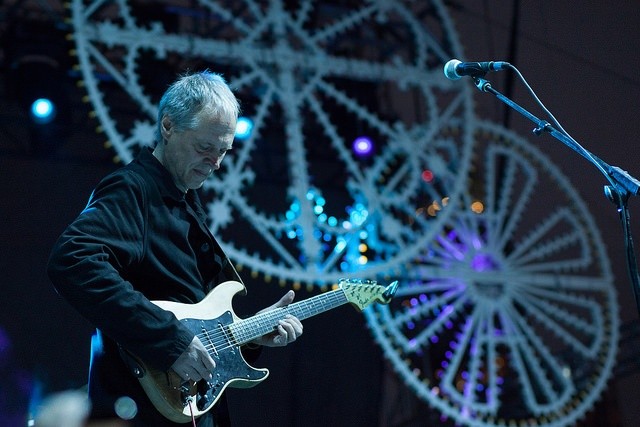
[116,278,399,425]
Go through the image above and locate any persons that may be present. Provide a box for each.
[47,69,304,427]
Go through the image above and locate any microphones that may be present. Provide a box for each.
[444,59,510,80]
